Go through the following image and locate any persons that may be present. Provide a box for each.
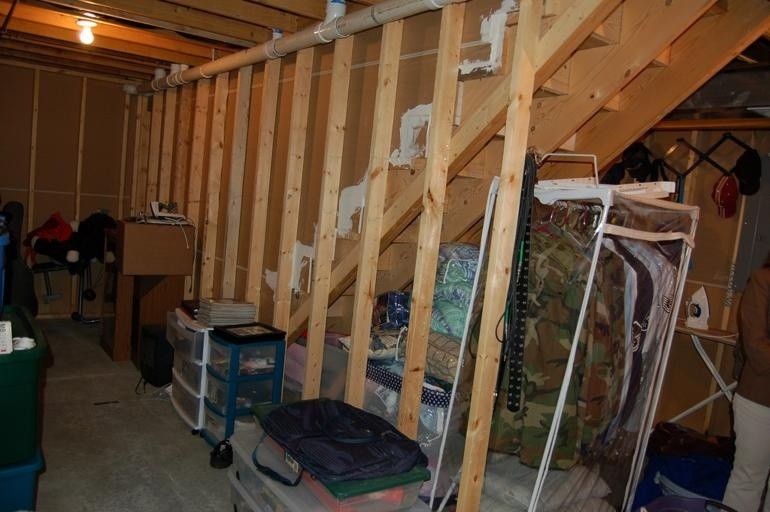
[722,268,770,512]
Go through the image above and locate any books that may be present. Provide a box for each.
[197,297,256,326]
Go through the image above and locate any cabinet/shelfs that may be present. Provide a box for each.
[167,311,287,454]
[99,268,200,364]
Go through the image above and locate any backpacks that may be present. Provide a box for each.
[251,398,428,486]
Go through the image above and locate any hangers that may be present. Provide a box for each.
[495,195,683,298]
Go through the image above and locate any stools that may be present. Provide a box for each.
[31,244,101,328]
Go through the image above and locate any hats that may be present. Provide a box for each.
[601,142,651,184]
[712,150,762,218]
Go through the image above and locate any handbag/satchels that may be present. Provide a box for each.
[135,329,174,395]
[631,421,736,511]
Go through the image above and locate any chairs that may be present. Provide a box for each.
[0,199,39,320]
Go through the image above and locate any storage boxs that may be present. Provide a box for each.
[1,213,53,511]
[225,391,440,512]
[114,218,196,278]
